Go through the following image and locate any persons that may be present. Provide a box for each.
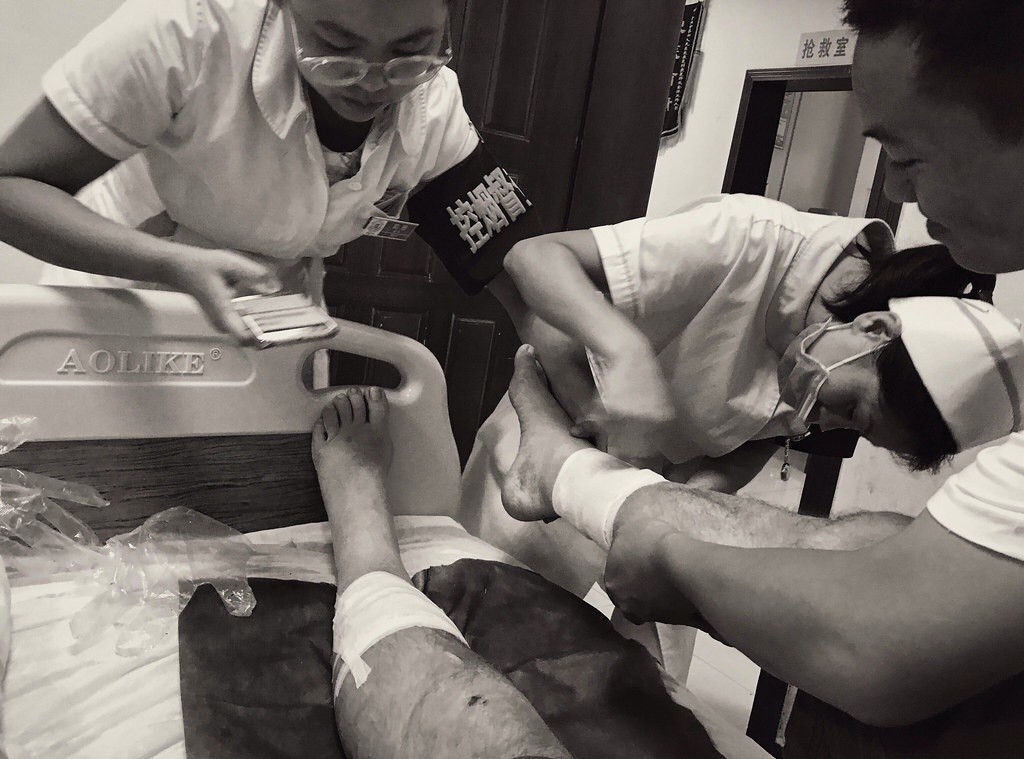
[458,195,1023,686]
[606,0,1023,724]
[0,0,604,439]
[312,344,917,759]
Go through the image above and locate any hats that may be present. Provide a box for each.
[888,296,1024,451]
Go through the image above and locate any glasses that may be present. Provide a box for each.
[287,2,453,88]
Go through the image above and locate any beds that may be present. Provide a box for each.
[0,283,777,759]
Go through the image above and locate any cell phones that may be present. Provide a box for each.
[232,291,339,352]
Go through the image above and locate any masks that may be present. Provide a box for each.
[777,316,891,422]
